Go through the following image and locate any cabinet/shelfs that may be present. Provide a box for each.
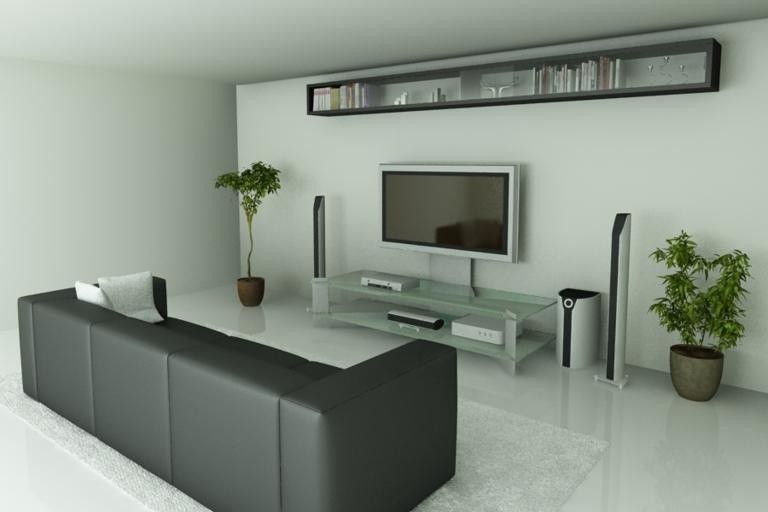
[306,38,722,120]
[312,269,557,375]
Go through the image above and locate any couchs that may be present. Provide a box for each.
[18,275,456,510]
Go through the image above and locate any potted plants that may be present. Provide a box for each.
[646,229,755,402]
[214,161,282,306]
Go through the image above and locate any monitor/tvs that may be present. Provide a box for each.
[377,161,521,264]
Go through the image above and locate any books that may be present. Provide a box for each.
[310,84,363,112]
[530,54,625,96]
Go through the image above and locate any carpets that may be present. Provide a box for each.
[5,394,611,510]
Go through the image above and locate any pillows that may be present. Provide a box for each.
[74,272,166,327]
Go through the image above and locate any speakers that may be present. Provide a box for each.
[555,288,602,370]
[594,211,633,390]
[311,194,328,278]
